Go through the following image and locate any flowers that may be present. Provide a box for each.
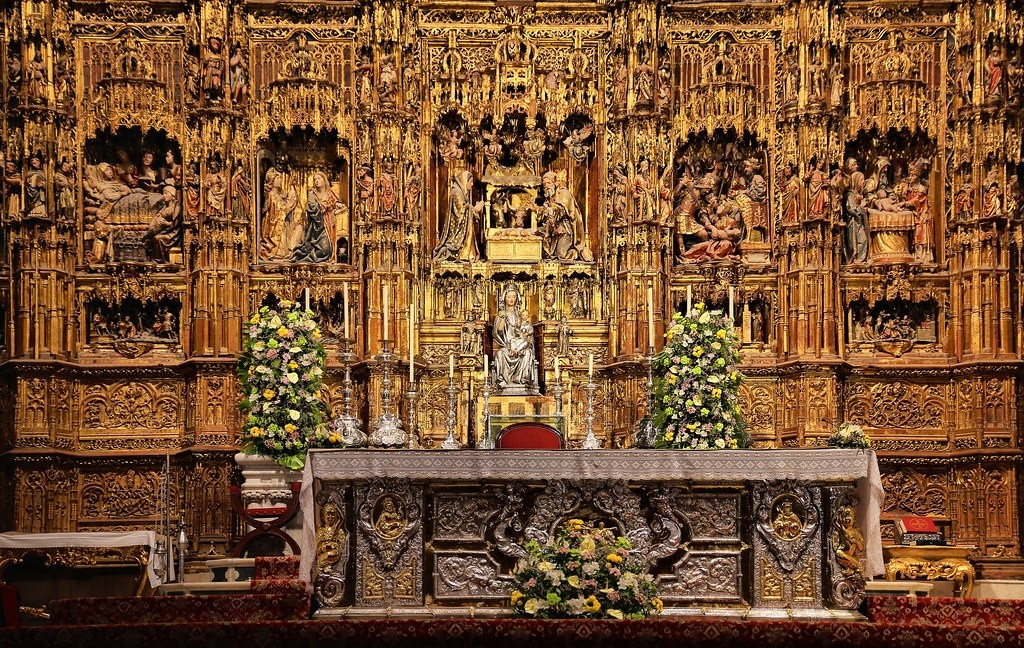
[829,422,872,451]
[236,300,343,470]
[508,519,663,620]
[649,302,753,451]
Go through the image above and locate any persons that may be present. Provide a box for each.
[352,159,418,220]
[784,54,844,108]
[4,143,249,269]
[957,45,1024,110]
[435,275,484,355]
[751,304,764,342]
[492,283,540,395]
[834,504,864,576]
[6,49,70,108]
[262,152,345,266]
[544,278,593,356]
[613,49,670,116]
[955,169,1020,221]
[774,503,801,539]
[316,501,345,575]
[855,302,930,339]
[185,39,250,109]
[431,167,481,264]
[86,301,177,344]
[614,138,933,264]
[528,171,584,263]
[377,500,403,537]
[358,48,414,111]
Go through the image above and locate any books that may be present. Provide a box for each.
[895,516,938,535]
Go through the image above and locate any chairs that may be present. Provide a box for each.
[495,422,566,450]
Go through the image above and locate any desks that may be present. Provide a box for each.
[882,546,979,597]
[297,448,886,621]
[0,531,175,596]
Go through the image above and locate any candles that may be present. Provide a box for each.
[450,354,453,378]
[484,354,488,375]
[555,357,559,379]
[589,354,593,376]
[305,289,310,310]
[728,286,733,318]
[409,304,415,382]
[344,284,349,337]
[649,288,653,346]
[383,285,388,339]
[687,285,692,314]
